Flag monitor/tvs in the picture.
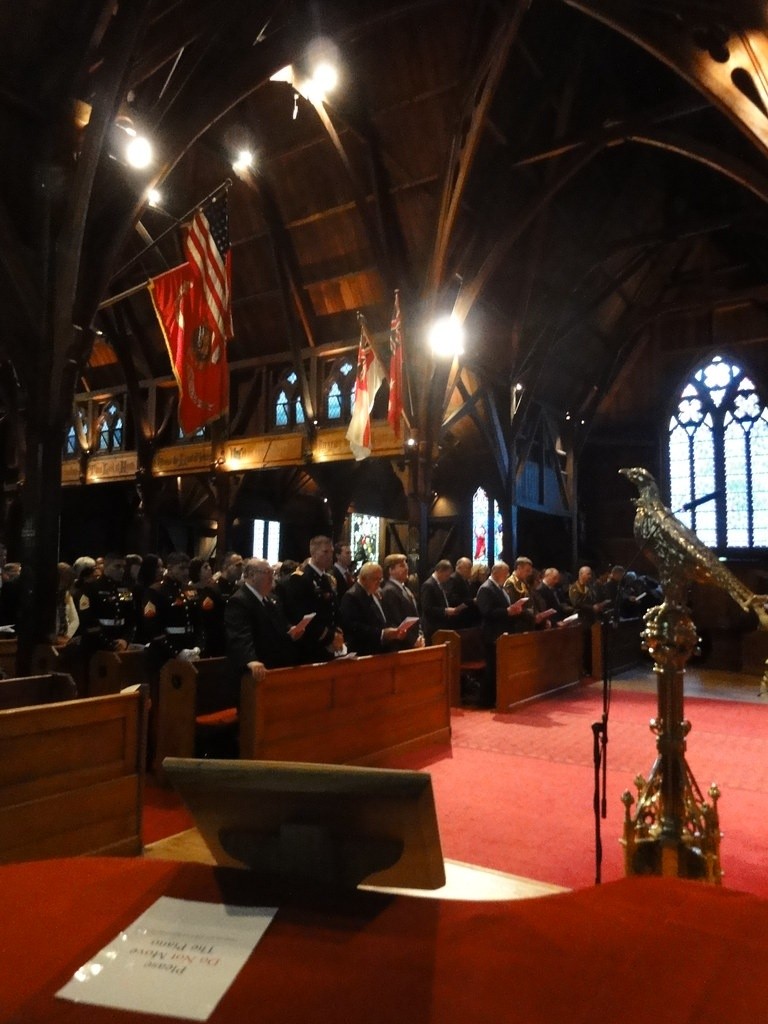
[162,755,447,891]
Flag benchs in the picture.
[0,626,500,786]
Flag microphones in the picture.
[613,489,724,628]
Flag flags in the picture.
[182,185,235,345]
[346,323,387,461]
[386,293,407,439]
[150,257,229,438]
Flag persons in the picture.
[1,534,676,727]
